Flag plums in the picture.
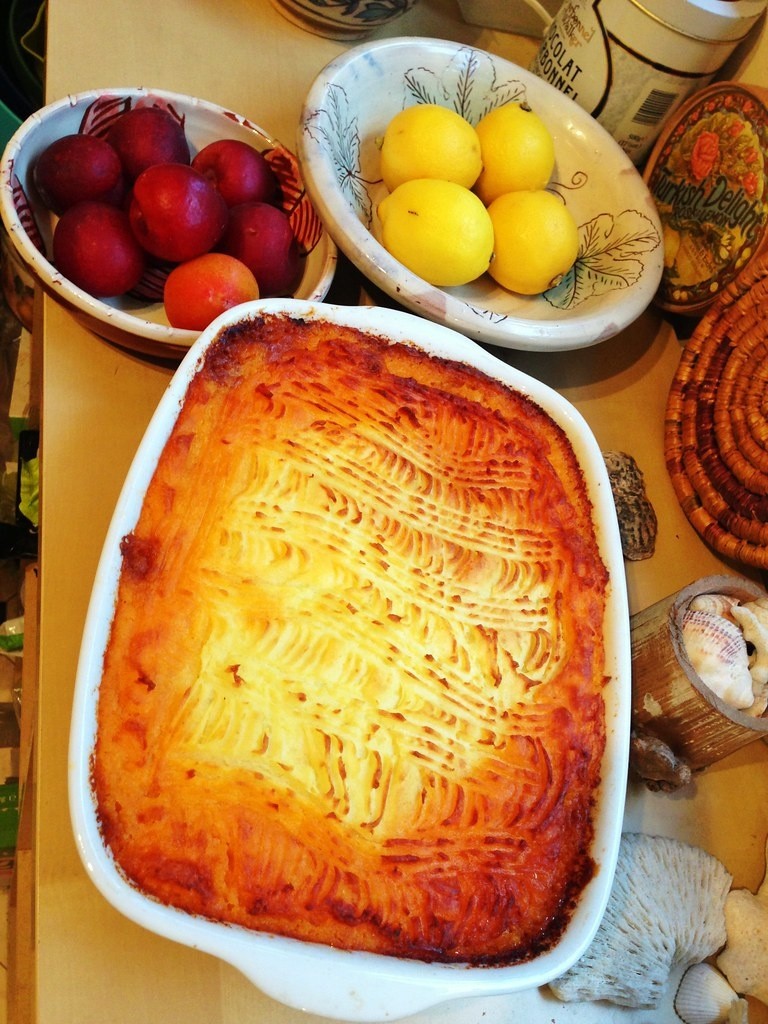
[22,105,296,335]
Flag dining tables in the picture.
[8,0,767,1024]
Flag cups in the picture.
[634,576,768,787]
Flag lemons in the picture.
[371,101,581,292]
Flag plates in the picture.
[63,302,633,1024]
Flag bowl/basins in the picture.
[3,85,336,356]
[305,33,662,350]
